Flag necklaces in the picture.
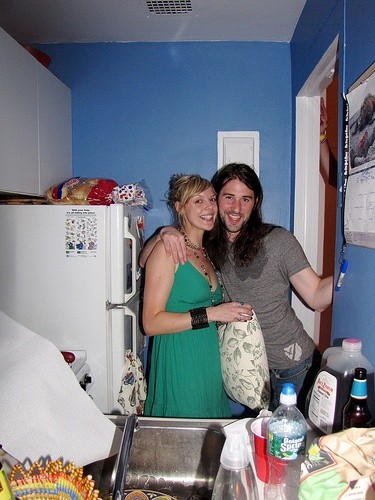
[177,226,225,331]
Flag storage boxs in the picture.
[23,43,53,69]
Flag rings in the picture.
[240,301,244,306]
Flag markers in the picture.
[334,259,349,291]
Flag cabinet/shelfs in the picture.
[0,25,75,200]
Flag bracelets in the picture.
[189,306,210,330]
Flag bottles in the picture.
[265,382,307,500]
[308,339,375,439]
[212,431,260,500]
[342,368,372,432]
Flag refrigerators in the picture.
[0,203,146,417]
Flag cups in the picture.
[250,417,302,485]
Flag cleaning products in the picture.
[212,431,260,500]
[263,382,309,499]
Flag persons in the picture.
[142,173,253,418]
[138,162,335,419]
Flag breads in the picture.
[45,179,98,205]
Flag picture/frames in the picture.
[216,130,259,178]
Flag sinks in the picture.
[83,417,126,500]
[110,416,236,500]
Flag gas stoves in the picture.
[60,350,96,395]
